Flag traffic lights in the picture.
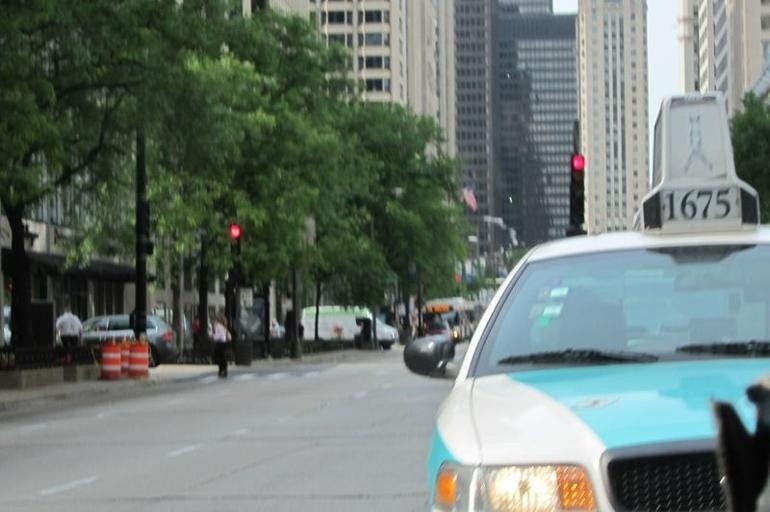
[570,154,584,226]
[227,221,242,266]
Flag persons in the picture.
[443,305,457,332]
[190,314,200,349]
[207,311,234,378]
[56,304,85,362]
[299,323,304,347]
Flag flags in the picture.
[463,184,479,213]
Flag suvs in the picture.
[56,310,180,370]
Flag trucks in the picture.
[298,303,399,351]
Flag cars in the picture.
[403,92,769,512]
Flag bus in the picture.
[419,295,478,344]
